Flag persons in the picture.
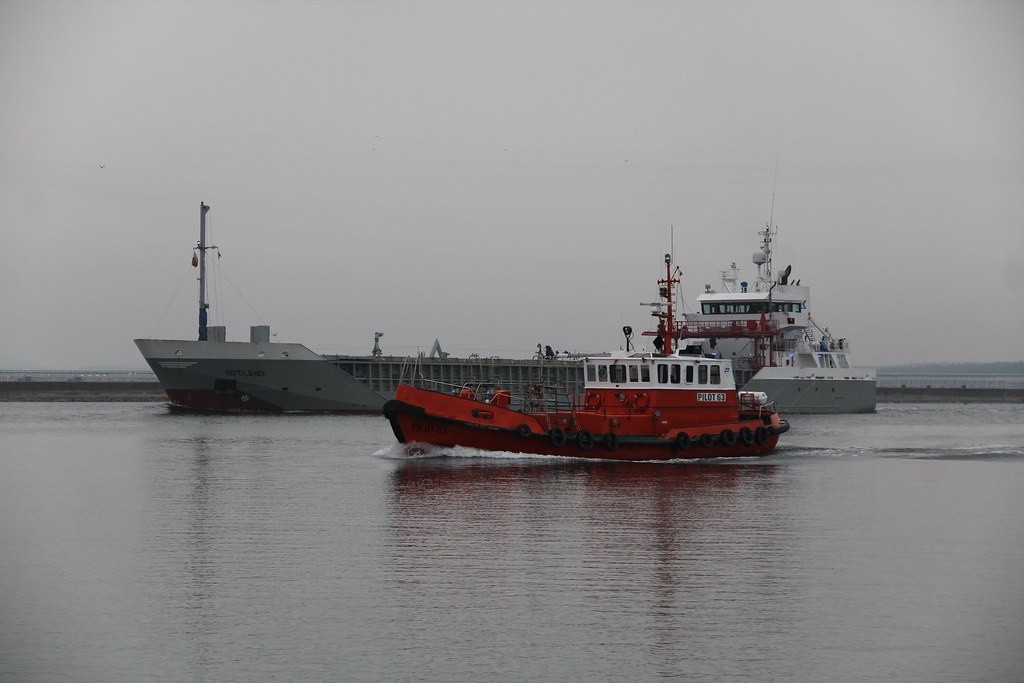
[820,336,846,350]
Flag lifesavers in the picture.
[583,392,602,410]
[632,392,650,412]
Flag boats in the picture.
[379,356,790,459]
[133,224,880,416]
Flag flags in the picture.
[192,252,198,268]
